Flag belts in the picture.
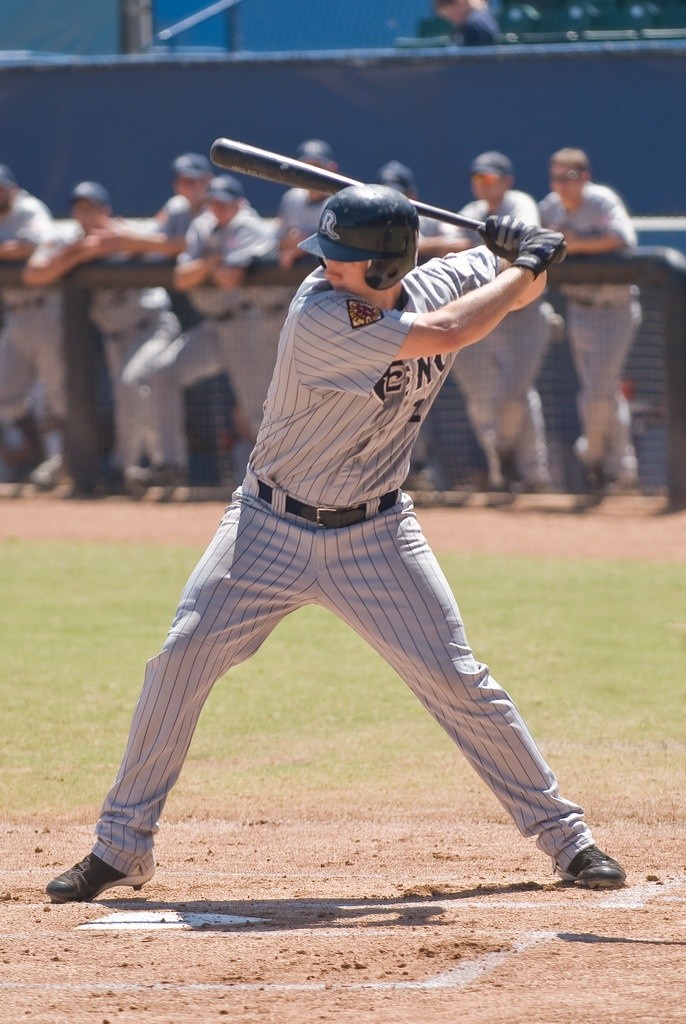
[257,480,399,530]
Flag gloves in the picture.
[477,215,529,263]
[512,224,564,282]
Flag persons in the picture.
[538,149,641,495]
[24,180,189,486]
[0,163,67,487]
[376,159,472,257]
[434,0,501,48]
[146,152,212,262]
[450,150,557,490]
[46,183,626,903]
[278,137,339,268]
[173,174,289,439]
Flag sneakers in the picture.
[45,852,155,903]
[557,846,628,887]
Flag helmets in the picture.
[68,180,110,205]
[297,184,420,290]
[469,151,514,180]
[171,153,211,180]
[377,160,416,194]
[204,173,245,202]
[293,139,336,167]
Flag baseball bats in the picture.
[210,137,568,264]
